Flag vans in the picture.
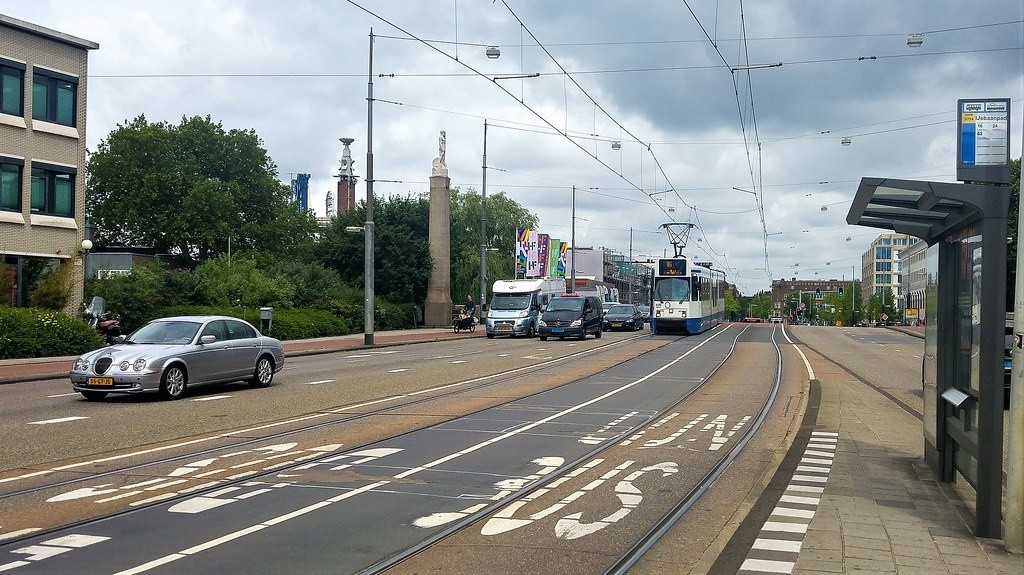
[538,293,604,341]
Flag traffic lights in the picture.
[815,287,821,296]
[796,308,804,317]
[838,287,844,296]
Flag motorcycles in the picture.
[81,296,122,344]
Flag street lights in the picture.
[345,222,374,345]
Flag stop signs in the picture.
[838,306,842,310]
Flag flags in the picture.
[515,227,568,279]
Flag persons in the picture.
[458,294,476,329]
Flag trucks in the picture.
[482,278,567,338]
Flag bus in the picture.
[649,258,726,336]
[743,318,761,323]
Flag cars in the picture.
[638,305,651,323]
[791,320,811,326]
[603,304,644,331]
[68,315,286,401]
[601,302,621,316]
[921,319,1014,411]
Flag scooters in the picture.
[452,308,478,333]
